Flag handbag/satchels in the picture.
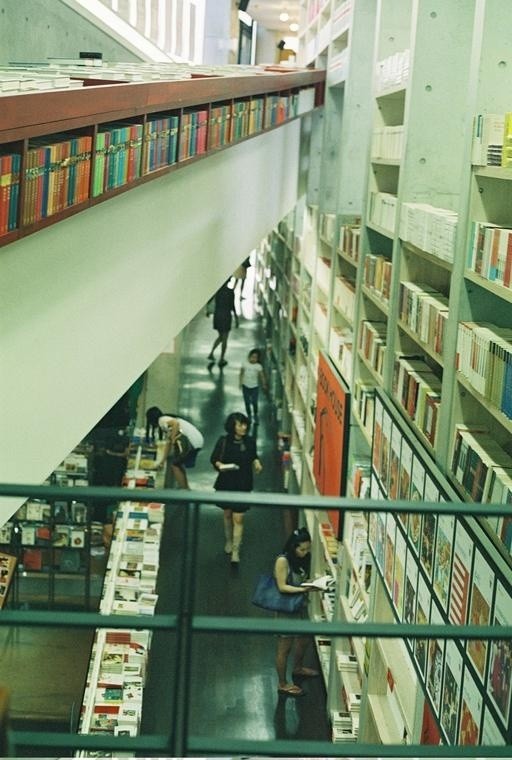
[207,298,218,314]
[251,573,309,614]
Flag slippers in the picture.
[278,682,305,697]
[293,668,321,677]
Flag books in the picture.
[0,428,166,757]
[258,111,511,746]
[297,0,410,90]
[0,59,325,234]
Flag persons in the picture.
[206,278,239,366]
[210,410,321,697]
[146,409,203,490]
[233,256,250,299]
[239,349,266,424]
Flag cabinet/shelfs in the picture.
[0,444,92,612]
[0,65,326,247]
